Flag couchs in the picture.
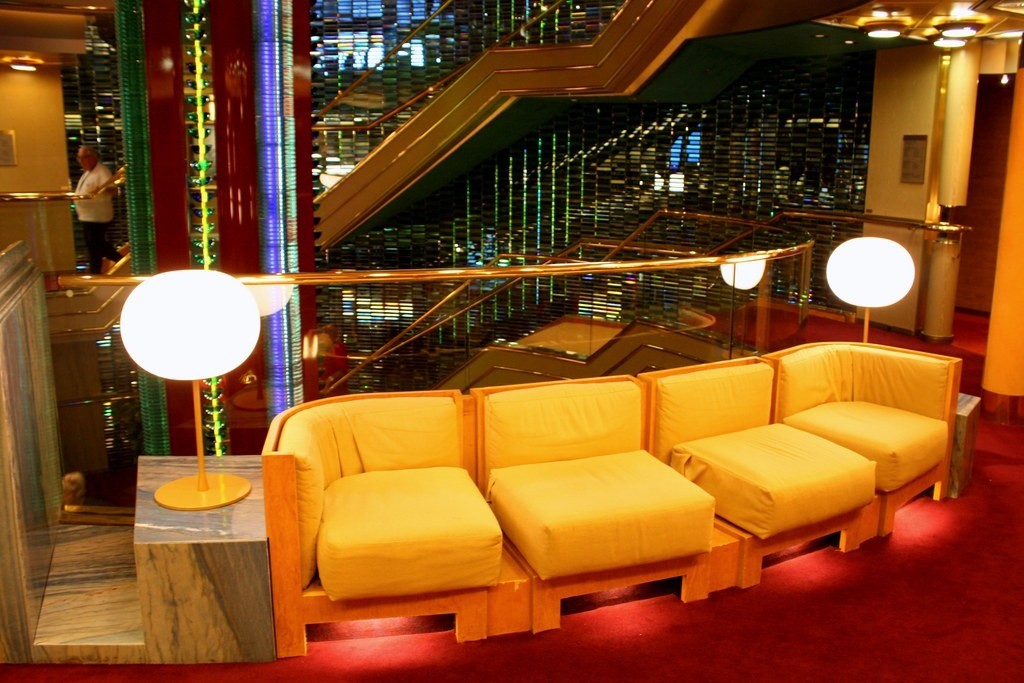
[261,340,963,659]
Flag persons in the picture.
[73,144,123,276]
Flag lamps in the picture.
[826,236,915,344]
[119,269,261,511]
[720,259,768,355]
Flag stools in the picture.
[948,393,982,498]
